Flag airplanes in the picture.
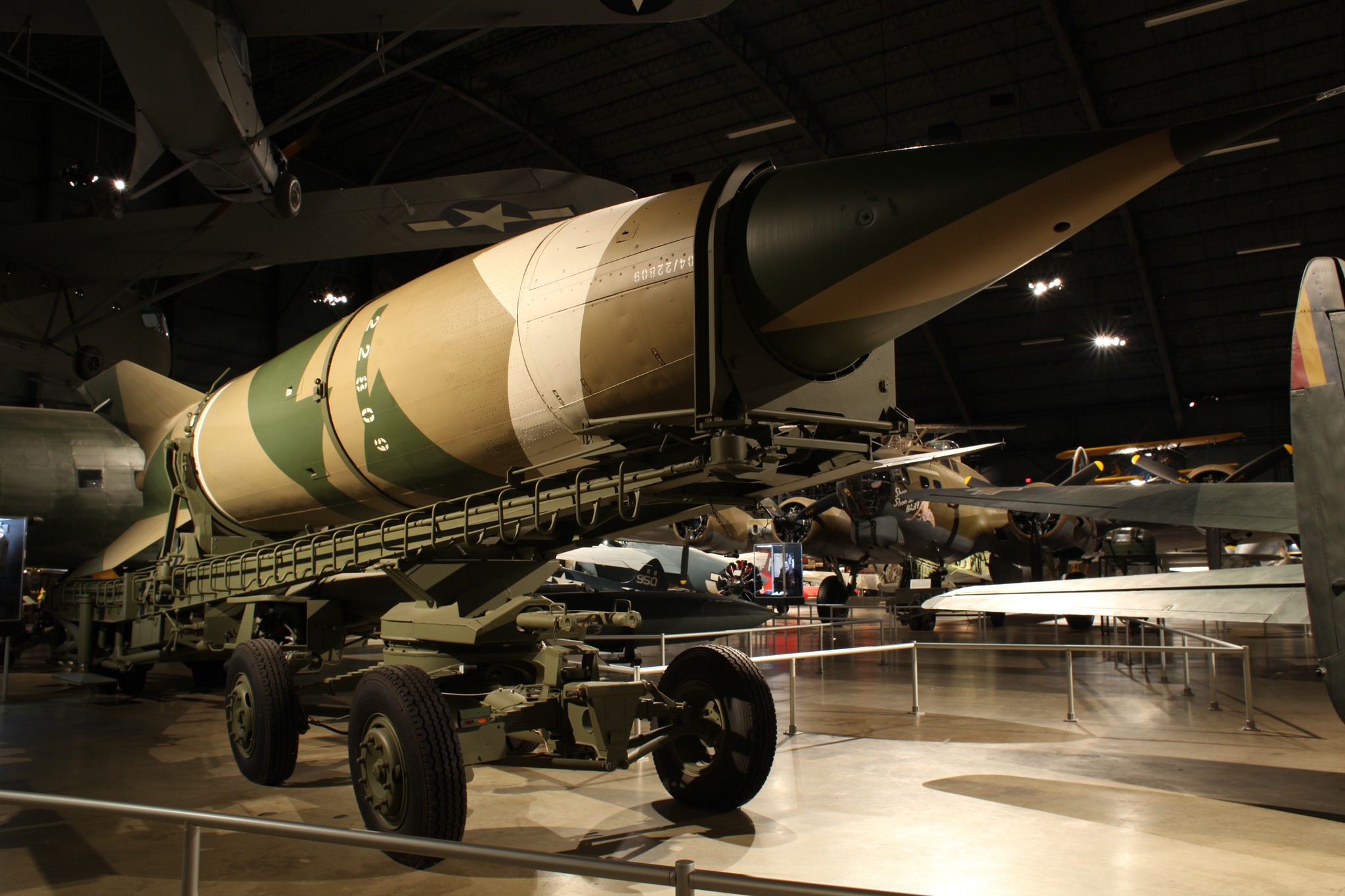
[0,0,1345,873]
